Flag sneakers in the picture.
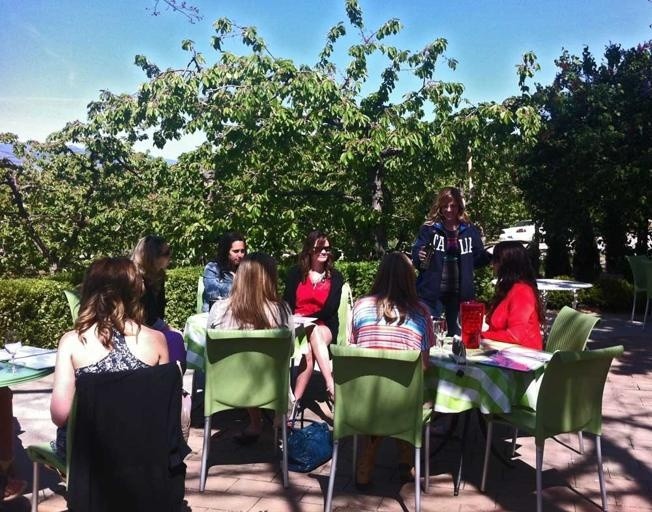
[233,416,283,463]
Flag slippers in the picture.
[0,479,27,502]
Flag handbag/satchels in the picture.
[449,334,466,365]
[287,422,332,473]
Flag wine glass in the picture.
[3,334,22,376]
[432,320,448,353]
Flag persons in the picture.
[451,240,546,438]
[278,230,346,429]
[409,186,493,338]
[129,234,186,374]
[209,253,294,437]
[346,249,438,493]
[2,387,30,505]
[199,231,251,310]
[47,253,171,467]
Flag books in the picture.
[1,343,60,372]
[482,343,552,375]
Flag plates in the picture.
[498,238,531,244]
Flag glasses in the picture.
[313,247,330,251]
[159,247,173,258]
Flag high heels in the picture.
[325,388,335,413]
[288,399,301,429]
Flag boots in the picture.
[356,435,381,484]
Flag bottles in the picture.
[420,231,436,270]
